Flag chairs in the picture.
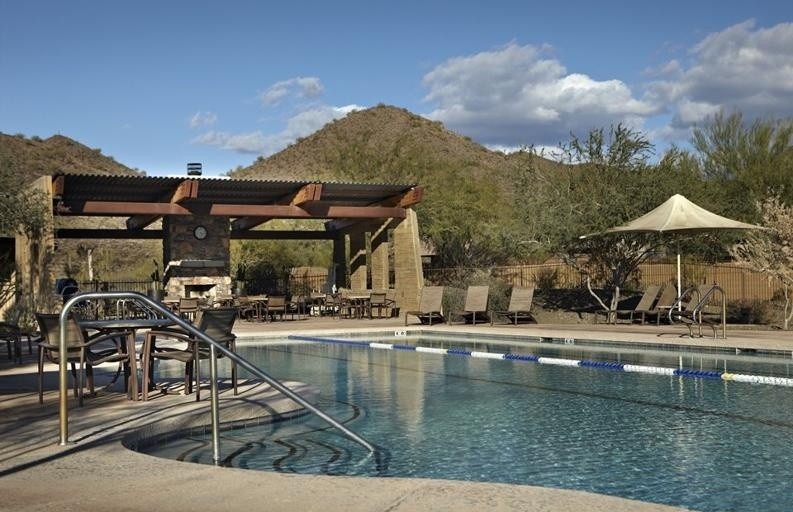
[138,305,242,403]
[447,285,492,329]
[33,310,134,406]
[56,278,87,307]
[2,287,45,365]
[594,286,713,341]
[403,285,448,327]
[494,284,539,328]
[114,290,399,324]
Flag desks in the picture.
[81,316,179,400]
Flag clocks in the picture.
[193,226,208,240]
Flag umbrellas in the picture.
[578,193,771,322]
